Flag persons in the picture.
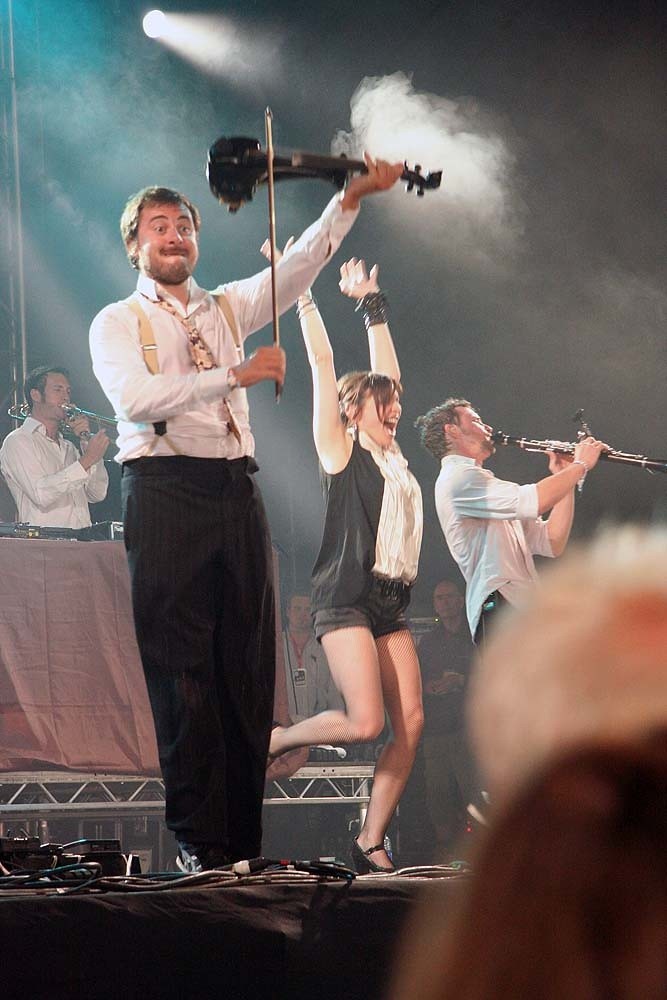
[88,152,405,878]
[243,235,428,874]
[0,367,111,529]
[284,396,667,1000]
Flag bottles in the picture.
[384,835,393,862]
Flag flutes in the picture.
[492,427,667,471]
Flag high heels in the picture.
[351,836,395,874]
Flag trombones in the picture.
[5,399,118,432]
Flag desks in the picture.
[0,540,308,783]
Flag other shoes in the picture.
[175,842,233,876]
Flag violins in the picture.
[207,134,446,215]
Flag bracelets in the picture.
[352,293,388,330]
[572,461,587,492]
[295,295,318,318]
